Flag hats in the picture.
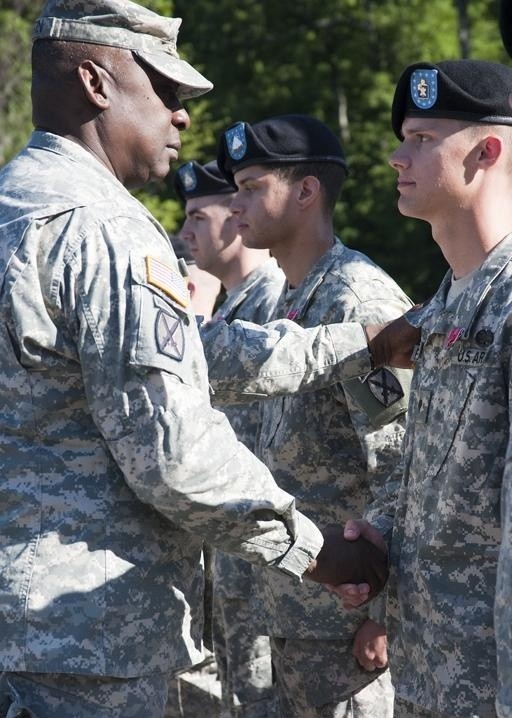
[392,60,512,142]
[33,0,214,100]
[174,114,345,202]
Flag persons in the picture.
[0,0,423,718]
[167,115,416,718]
[322,59,512,718]
